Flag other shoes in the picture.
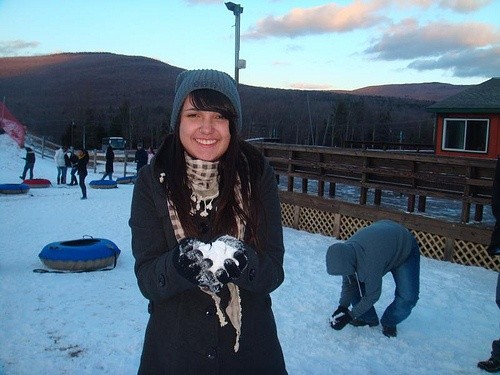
[383,324,398,339]
[81,196,86,200]
[73,183,78,186]
[20,176,24,180]
[67,183,74,186]
[351,316,377,327]
[479,357,500,372]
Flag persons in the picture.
[134,143,148,176]
[148,146,155,165]
[20,147,35,180]
[101,147,114,180]
[326,219,420,338]
[77,148,89,199]
[477,159,500,373]
[54,145,70,184]
[70,153,79,184]
[129,70,289,375]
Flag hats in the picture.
[170,68,241,136]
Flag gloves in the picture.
[330,306,350,329]
[174,239,213,285]
[216,253,246,284]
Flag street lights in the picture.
[224,1,246,91]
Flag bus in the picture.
[102,136,127,153]
